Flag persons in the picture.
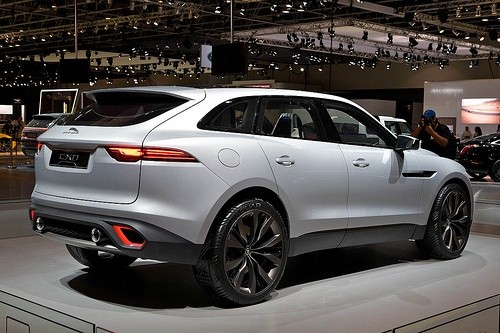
[413,110,456,161]
[461,127,473,140]
[2,116,24,138]
[473,127,482,138]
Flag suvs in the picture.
[28,86,474,306]
[21,114,61,158]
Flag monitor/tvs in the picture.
[201,45,212,68]
[59,58,89,83]
[212,42,248,76]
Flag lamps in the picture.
[0,0,500,92]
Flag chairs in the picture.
[237,112,305,140]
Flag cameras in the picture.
[422,118,432,126]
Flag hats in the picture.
[421,110,436,118]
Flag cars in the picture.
[457,132,500,183]
